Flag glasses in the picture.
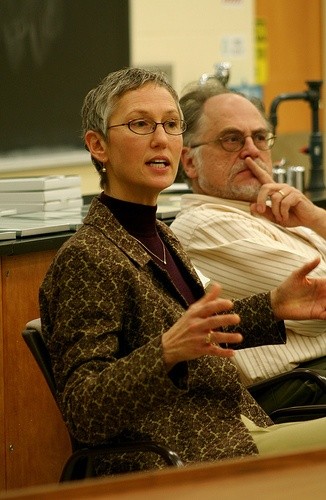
[191,131,277,153]
[107,117,190,136]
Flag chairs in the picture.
[21,315,185,479]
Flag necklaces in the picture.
[125,224,167,265]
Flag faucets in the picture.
[267,79,325,191]
[198,63,231,89]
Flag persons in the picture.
[38,67,326,478]
[168,81,326,423]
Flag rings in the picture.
[278,190,285,198]
[205,330,215,345]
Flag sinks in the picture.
[81,193,182,214]
